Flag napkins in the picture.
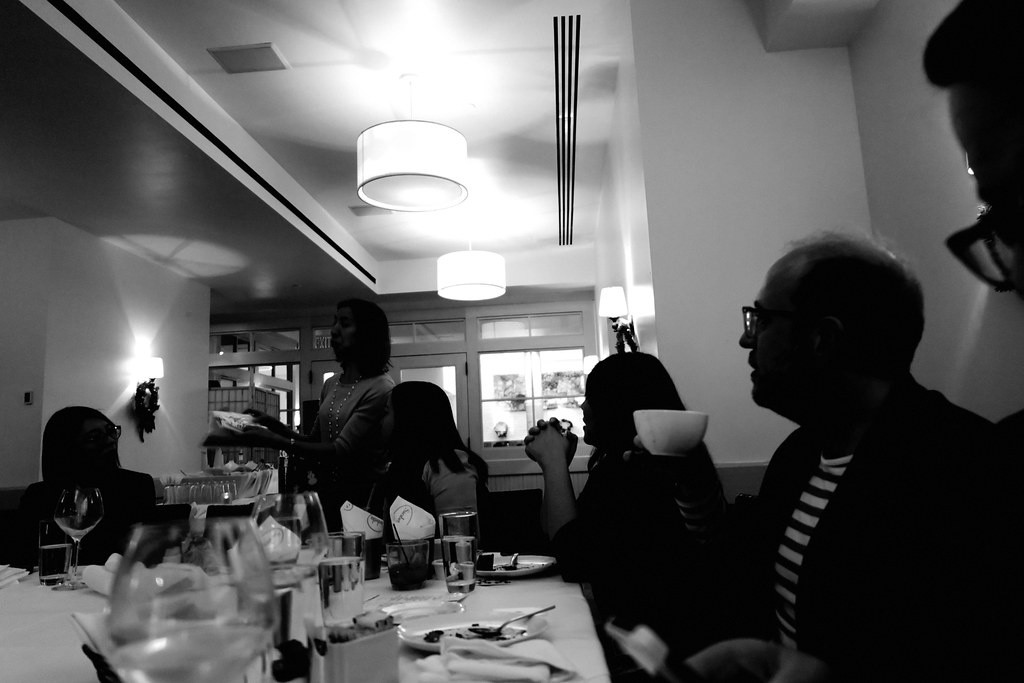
[0,563,30,590]
[413,636,581,682]
[71,583,238,659]
[258,515,301,563]
[84,553,124,597]
[389,495,436,540]
[340,500,384,540]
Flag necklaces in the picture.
[328,375,362,438]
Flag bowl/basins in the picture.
[633,409,709,457]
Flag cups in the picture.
[39,516,73,587]
[439,511,479,594]
[310,531,366,635]
[385,540,430,591]
[164,479,240,504]
[251,491,332,590]
[321,557,366,633]
[101,516,280,683]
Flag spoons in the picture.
[502,553,519,571]
[467,604,556,636]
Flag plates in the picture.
[476,556,555,579]
[382,601,463,625]
[398,610,547,652]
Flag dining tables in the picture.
[0,567,610,683]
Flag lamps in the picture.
[356,73,470,212]
[437,239,506,301]
[598,285,634,337]
[133,357,163,415]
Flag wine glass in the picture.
[52,485,104,591]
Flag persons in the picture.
[525,352,731,670]
[0,406,156,572]
[623,233,995,667]
[381,382,489,543]
[686,0,1024,683]
[236,299,396,532]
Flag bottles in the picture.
[237,453,245,466]
[252,459,264,472]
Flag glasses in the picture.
[742,306,823,338]
[74,425,122,446]
[946,202,1023,290]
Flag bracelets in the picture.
[285,438,296,456]
